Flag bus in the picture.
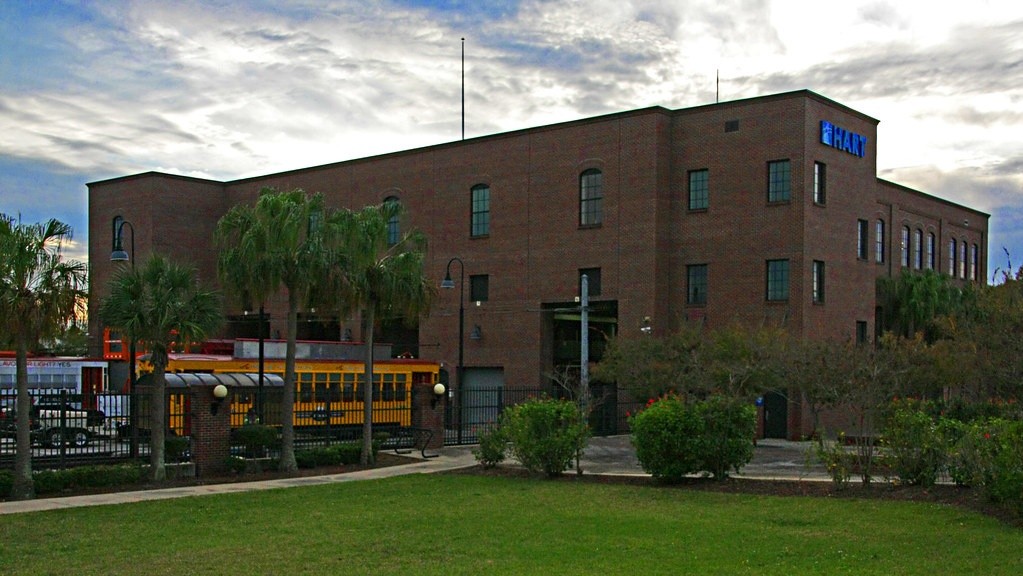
[129,337,442,443]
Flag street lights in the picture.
[440,257,473,445]
[109,221,139,461]
[575,274,588,424]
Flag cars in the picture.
[0,394,119,448]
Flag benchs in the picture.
[838,435,900,476]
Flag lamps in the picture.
[470,324,482,340]
[431,383,445,410]
[210,385,227,416]
